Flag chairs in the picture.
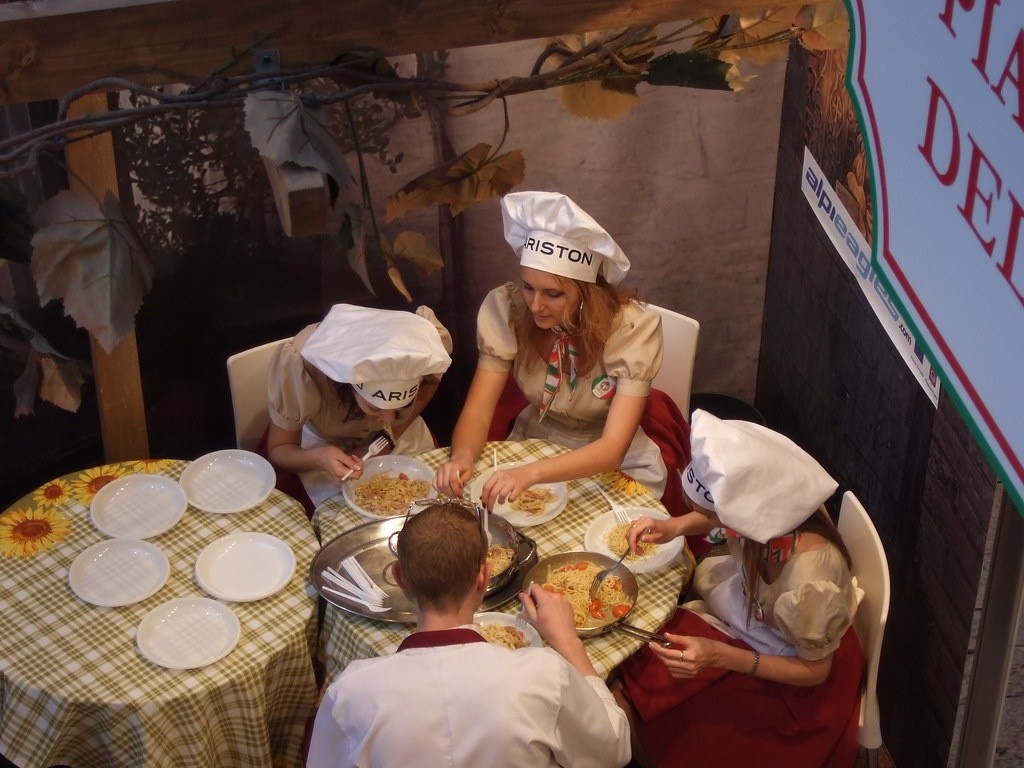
[836,490,891,768]
[225,336,295,452]
[625,296,699,424]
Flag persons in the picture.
[308,503,633,767]
[433,191,691,515]
[254,303,452,518]
[609,409,868,768]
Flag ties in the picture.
[703,527,797,574]
[538,324,579,424]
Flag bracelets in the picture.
[375,433,395,449]
[752,652,759,673]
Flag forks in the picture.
[482,505,492,548]
[342,436,388,481]
[596,484,629,523]
[590,533,644,600]
[322,556,392,612]
[517,579,534,629]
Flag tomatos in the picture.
[513,631,524,640]
[398,473,408,480]
[542,562,632,620]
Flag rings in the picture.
[633,524,637,528]
[681,650,682,660]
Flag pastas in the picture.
[541,560,622,626]
[511,488,553,516]
[478,622,532,650]
[354,473,429,516]
[485,543,513,577]
[607,520,658,561]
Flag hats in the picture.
[500,191,630,284]
[302,305,452,409]
[680,407,841,542]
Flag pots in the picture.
[522,552,672,650]
[388,515,536,595]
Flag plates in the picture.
[471,613,543,649]
[585,507,684,574]
[471,460,568,526]
[180,450,276,514]
[91,475,187,541]
[137,598,240,669]
[342,456,438,519]
[68,540,170,606]
[195,532,296,602]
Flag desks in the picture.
[0,458,322,768]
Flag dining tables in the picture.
[310,439,698,715]
